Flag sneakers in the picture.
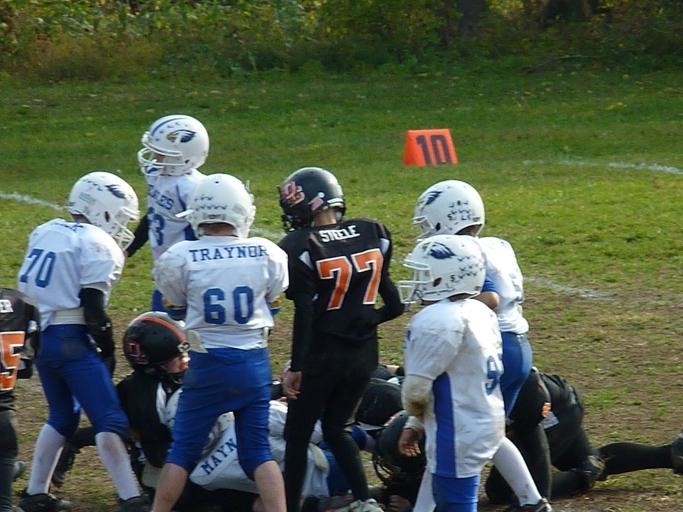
[111,492,151,512]
[332,497,384,511]
[669,433,683,474]
[575,455,607,490]
[496,496,554,512]
[15,481,74,510]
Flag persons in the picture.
[1,114,682,511]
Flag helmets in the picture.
[136,114,209,178]
[395,233,487,312]
[275,167,345,235]
[122,311,191,388]
[410,179,484,242]
[349,382,425,490]
[62,171,141,252]
[185,173,256,239]
